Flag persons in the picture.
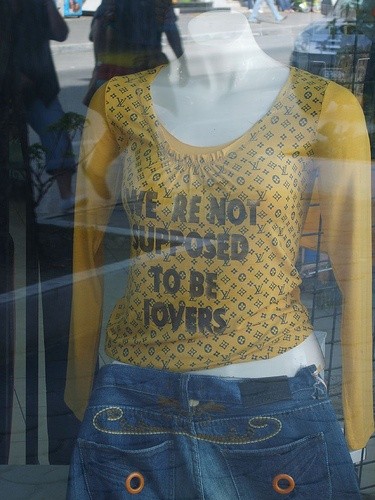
[84,0,190,123]
[0,0,80,216]
[248,0,296,23]
[65,11,373,500]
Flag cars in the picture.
[287,0,375,85]
